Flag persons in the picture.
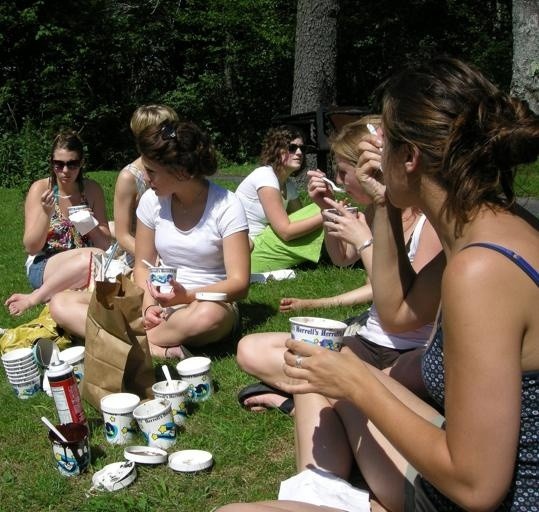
[234,124,352,274]
[4,133,112,316]
[101,103,255,272]
[48,120,249,361]
[212,57,539,511]
[234,113,444,419]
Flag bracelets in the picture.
[356,238,372,256]
[144,303,157,317]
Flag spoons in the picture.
[319,176,347,193]
[53,193,73,200]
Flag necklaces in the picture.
[173,184,208,215]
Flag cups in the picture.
[49,423,89,478]
[324,205,362,233]
[59,346,85,389]
[148,267,178,287]
[2,348,40,400]
[65,205,87,217]
[100,356,214,450]
[288,316,348,356]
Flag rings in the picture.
[295,356,302,369]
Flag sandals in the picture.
[237,381,294,419]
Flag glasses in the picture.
[160,118,185,153]
[286,144,308,156]
[51,159,81,171]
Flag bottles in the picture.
[46,346,92,443]
